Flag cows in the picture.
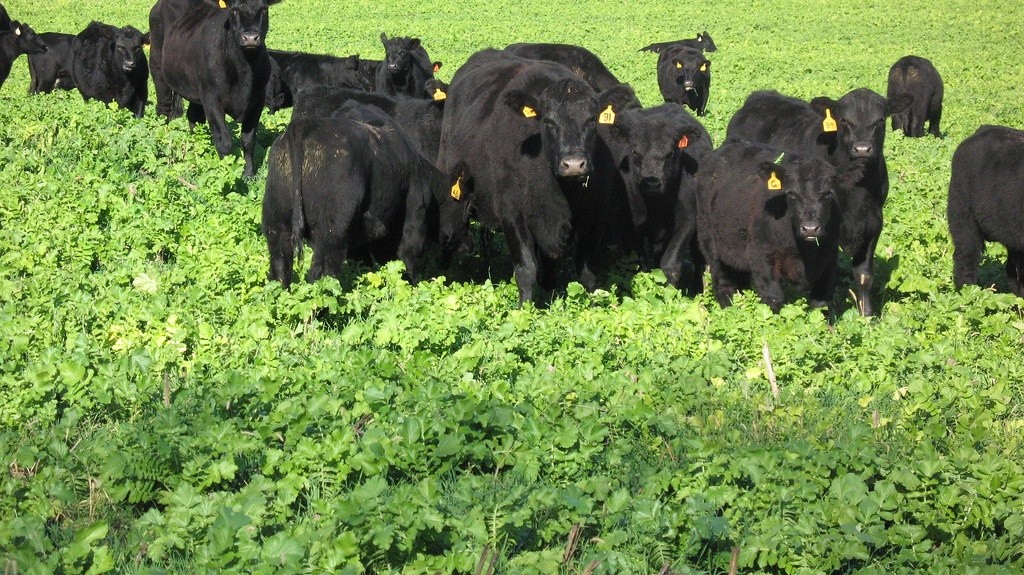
[945,123,1024,297]
[1,0,916,322]
[885,54,945,139]
